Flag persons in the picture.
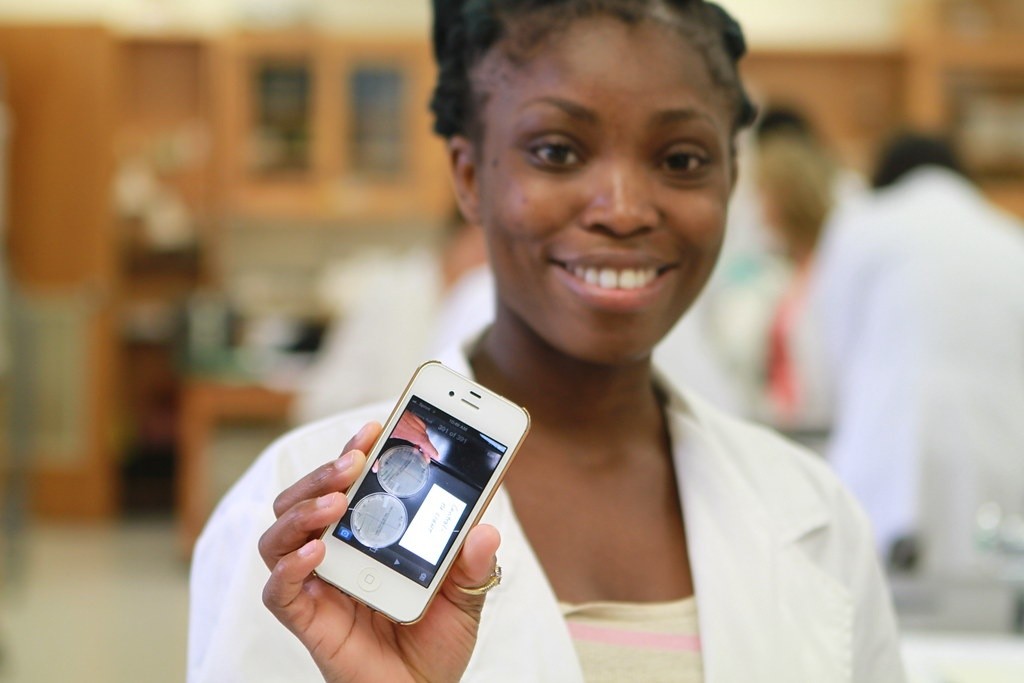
[188,1,908,683]
[287,84,1024,633]
[372,409,439,473]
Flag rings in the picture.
[447,566,503,596]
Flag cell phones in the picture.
[312,360,532,626]
